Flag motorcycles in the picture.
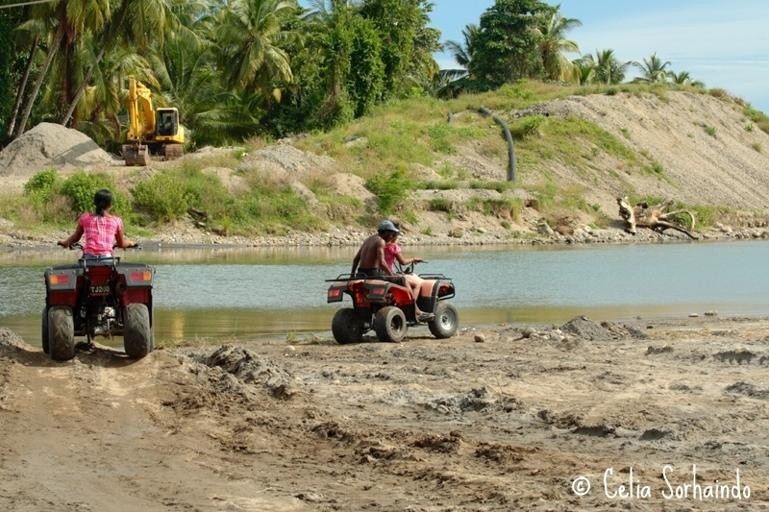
[324,259,458,342]
[41,241,153,359]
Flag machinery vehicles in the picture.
[121,76,184,165]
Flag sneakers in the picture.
[417,312,433,320]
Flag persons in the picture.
[159,116,172,135]
[57,189,137,330]
[381,228,425,303]
[352,221,433,321]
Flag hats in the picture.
[377,220,399,233]
